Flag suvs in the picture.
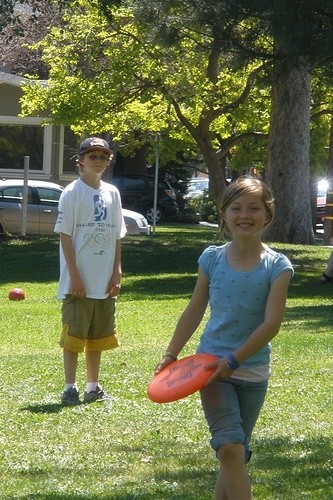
[110,174,178,224]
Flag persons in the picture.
[153,174,295,496]
[53,135,130,408]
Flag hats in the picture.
[80,137,113,154]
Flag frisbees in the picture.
[148,354,220,402]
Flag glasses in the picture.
[89,155,108,161]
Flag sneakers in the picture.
[84,385,113,403]
[62,386,81,405]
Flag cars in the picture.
[183,179,233,201]
[0,179,150,237]
[315,180,332,223]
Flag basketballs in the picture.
[8,289,25,301]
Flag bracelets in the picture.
[223,351,243,375]
[162,350,180,362]
